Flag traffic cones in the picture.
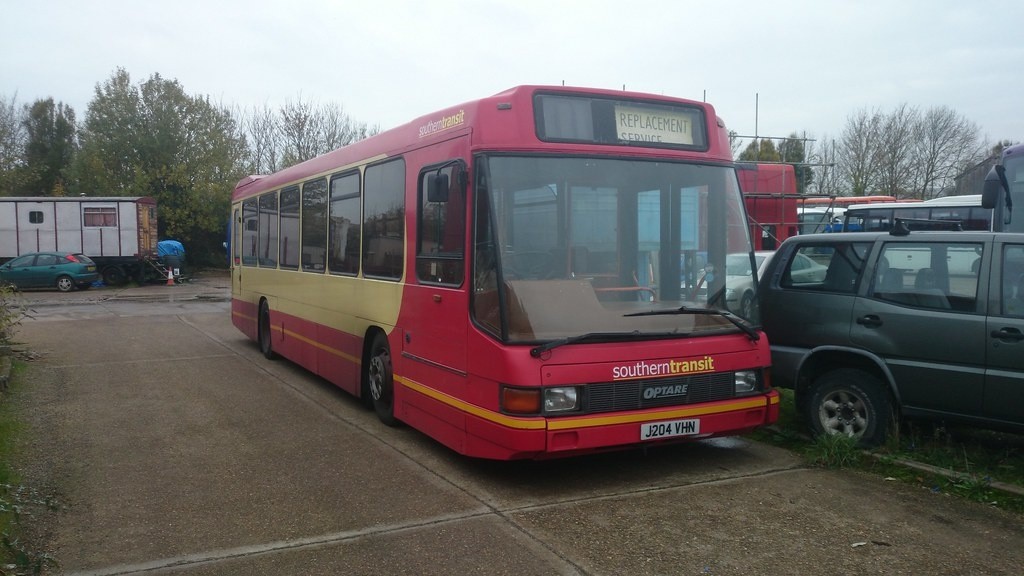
[165,266,176,285]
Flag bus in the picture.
[226,84,784,464]
[754,218,1024,450]
[798,196,925,208]
[849,195,994,232]
[797,207,847,234]
[981,143,1024,232]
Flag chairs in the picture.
[879,268,918,306]
[583,247,624,303]
[911,267,951,310]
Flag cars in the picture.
[679,251,830,322]
[0,251,97,291]
